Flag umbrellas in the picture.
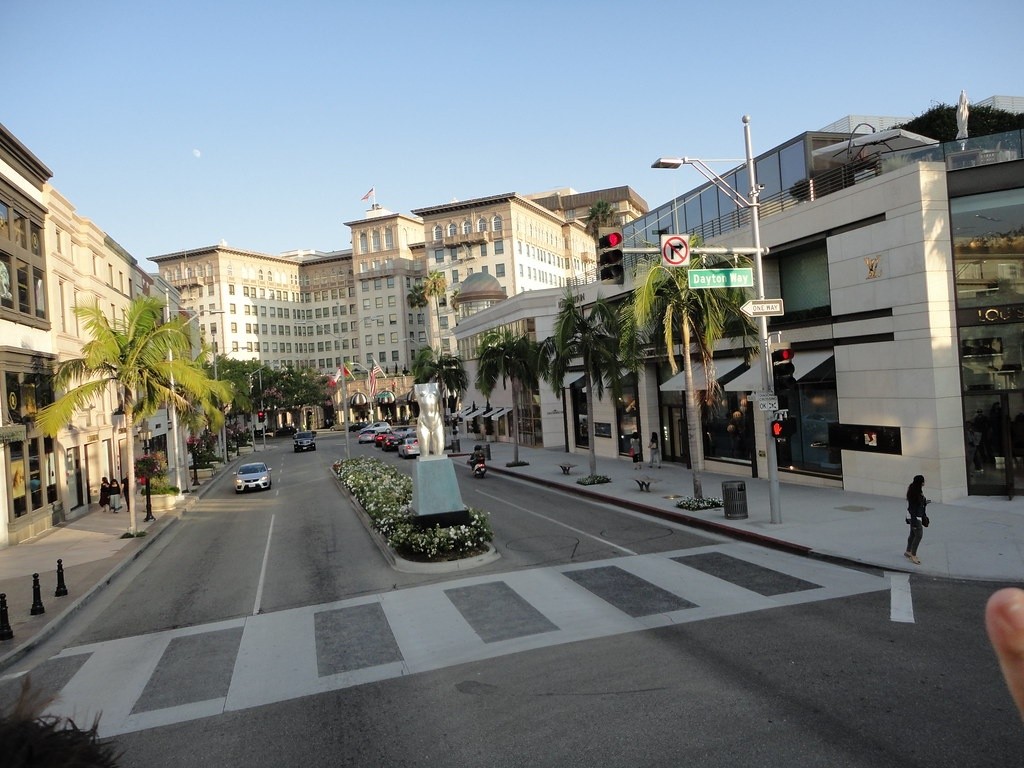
[811,128,939,178]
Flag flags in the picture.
[361,189,374,202]
[369,360,382,396]
[329,364,349,387]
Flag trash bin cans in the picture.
[452,439,460,453]
[482,444,491,460]
[722,481,748,520]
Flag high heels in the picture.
[904,551,920,564]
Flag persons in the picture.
[98,471,129,513]
[581,418,588,445]
[325,415,411,428]
[905,475,932,564]
[469,445,485,471]
[630,432,642,470]
[965,402,1024,474]
[984,588,1024,720]
[648,432,661,468]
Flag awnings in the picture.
[563,368,634,392]
[350,389,419,405]
[452,407,512,421]
[660,349,834,391]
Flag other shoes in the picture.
[633,468,636,470]
[648,466,652,468]
[657,466,661,469]
[974,469,983,472]
[639,464,641,470]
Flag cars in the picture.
[293,431,317,452]
[330,421,420,459]
[233,462,273,494]
[250,426,297,436]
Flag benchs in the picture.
[627,474,661,492]
[555,461,577,475]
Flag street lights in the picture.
[248,354,299,452]
[294,315,383,458]
[350,362,373,424]
[651,156,782,523]
[165,308,225,501]
[401,337,431,349]
[138,418,156,522]
[211,346,249,464]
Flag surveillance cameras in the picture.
[755,184,765,190]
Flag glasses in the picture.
[977,411,982,413]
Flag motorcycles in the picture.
[468,452,487,479]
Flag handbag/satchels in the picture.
[630,448,634,457]
[649,442,656,449]
[906,519,910,524]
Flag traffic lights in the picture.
[774,348,797,390]
[258,410,265,422]
[602,232,623,279]
[771,420,786,438]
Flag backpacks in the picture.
[970,431,982,445]
